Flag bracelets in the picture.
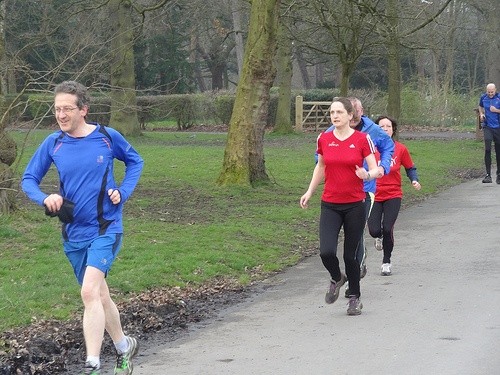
[367,171,371,180]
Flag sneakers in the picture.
[482,173,492,183]
[380,262,392,277]
[82,359,101,375]
[346,297,364,316]
[113,334,140,375]
[325,274,348,304]
[495,172,500,185]
[374,237,384,251]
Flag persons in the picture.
[478,82,500,185]
[20,81,143,375]
[300,98,376,316]
[367,114,421,276]
[315,97,395,279]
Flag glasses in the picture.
[55,106,80,112]
